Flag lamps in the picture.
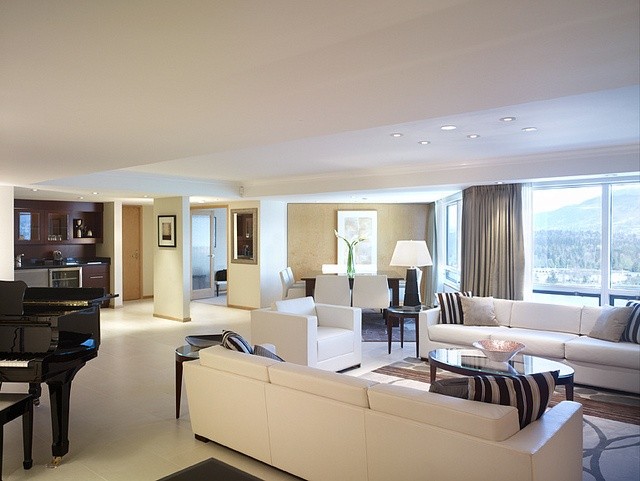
[388,240,434,307]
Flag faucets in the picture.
[16,254,24,264]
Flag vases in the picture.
[346,245,356,275]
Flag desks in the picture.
[385,305,433,358]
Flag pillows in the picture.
[427,377,468,400]
[459,296,501,326]
[467,369,561,431]
[253,344,286,364]
[434,291,475,325]
[619,301,640,345]
[587,303,635,343]
[221,330,254,355]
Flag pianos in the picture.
[0,280,119,468]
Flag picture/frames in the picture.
[157,215,177,247]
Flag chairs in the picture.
[214,270,227,298]
[351,274,391,309]
[279,266,306,298]
[313,274,352,307]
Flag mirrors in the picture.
[230,208,258,265]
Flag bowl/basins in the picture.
[472,339,525,362]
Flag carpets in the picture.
[356,355,640,481]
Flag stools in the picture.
[1,392,35,481]
[175,343,202,419]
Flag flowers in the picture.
[333,229,371,273]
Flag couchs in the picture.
[181,343,640,481]
[418,295,640,394]
[250,295,363,372]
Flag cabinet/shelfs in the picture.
[14,198,104,246]
[82,262,110,308]
